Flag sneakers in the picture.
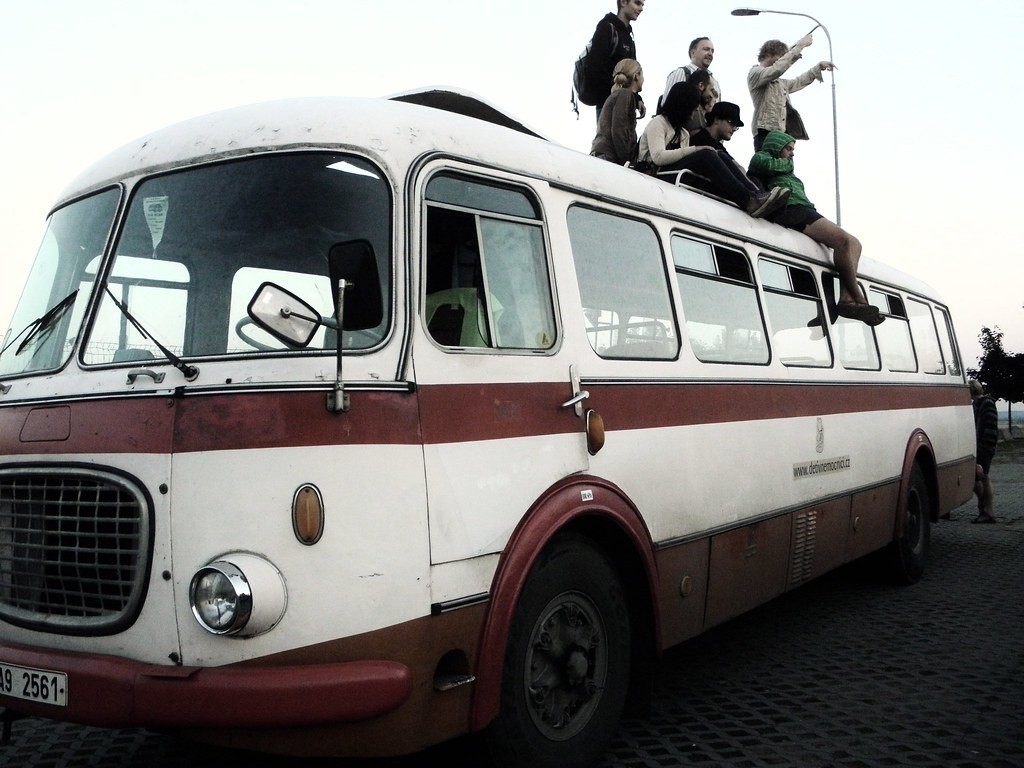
[759,187,791,218]
[746,186,781,218]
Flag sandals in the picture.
[864,314,885,326]
[971,512,996,523]
[836,301,879,322]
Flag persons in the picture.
[590,0,886,327]
[969,380,998,525]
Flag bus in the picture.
[0,89,976,768]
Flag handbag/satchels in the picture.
[633,162,657,177]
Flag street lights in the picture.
[731,8,841,229]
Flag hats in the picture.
[705,102,744,127]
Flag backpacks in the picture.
[573,22,619,106]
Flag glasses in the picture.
[725,118,740,128]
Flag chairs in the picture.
[424,285,488,347]
